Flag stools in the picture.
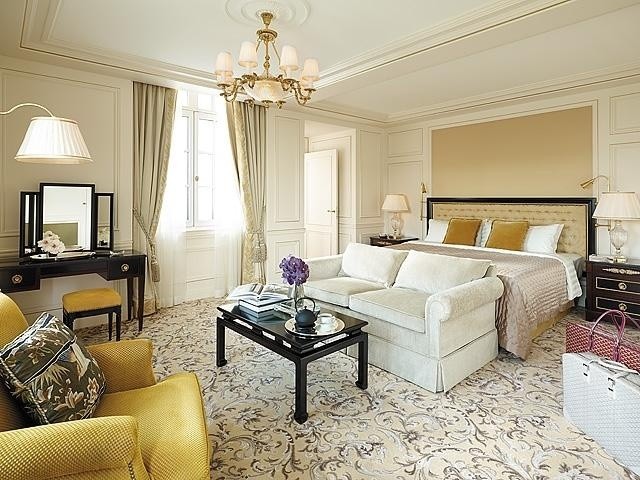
[61,288,123,341]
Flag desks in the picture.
[0,249,148,332]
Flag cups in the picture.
[389,235,393,239]
[396,235,400,239]
[320,313,335,324]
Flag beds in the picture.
[378,197,595,361]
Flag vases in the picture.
[287,284,304,307]
[49,252,58,257]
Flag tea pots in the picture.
[295,297,320,327]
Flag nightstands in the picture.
[583,257,640,329]
[370,236,419,247]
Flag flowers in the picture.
[279,254,309,286]
[43,230,61,240]
[38,238,65,254]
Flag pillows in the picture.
[424,218,565,254]
[0,310,107,425]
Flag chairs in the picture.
[0,293,213,480]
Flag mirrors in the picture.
[19,182,113,264]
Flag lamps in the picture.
[381,194,409,239]
[0,102,94,167]
[420,183,427,220]
[214,10,319,109]
[591,190,640,262]
[580,175,611,231]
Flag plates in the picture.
[379,235,387,239]
[285,316,345,336]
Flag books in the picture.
[225,280,293,323]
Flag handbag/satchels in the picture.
[565,309,639,373]
[562,351,640,475]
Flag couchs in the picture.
[300,240,505,395]
